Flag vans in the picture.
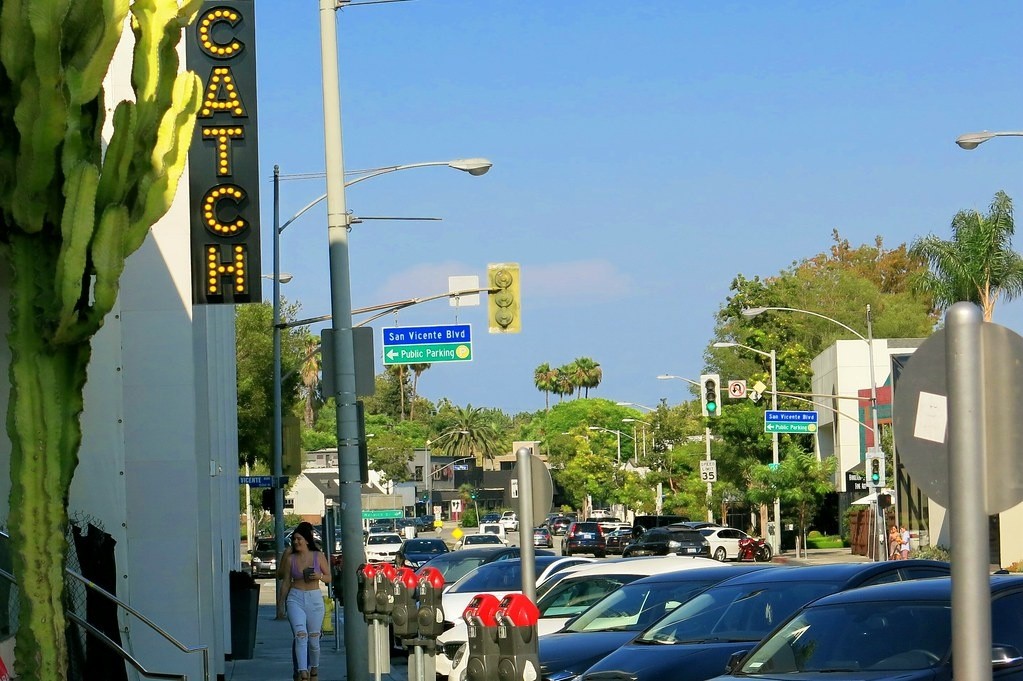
[475,524,507,541]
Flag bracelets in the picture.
[321,576,322,579]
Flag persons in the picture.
[276,522,332,681]
[889,525,910,560]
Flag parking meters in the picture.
[356,562,445,681]
[461,593,503,681]
[496,592,542,681]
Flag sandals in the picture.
[301,672,309,681]
[309,669,317,681]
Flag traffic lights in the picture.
[878,494,891,508]
[866,452,886,487]
[764,421,818,434]
[700,375,720,417]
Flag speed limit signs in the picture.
[699,460,717,482]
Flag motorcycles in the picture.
[736,533,772,561]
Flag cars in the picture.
[450,533,510,550]
[536,564,802,681]
[622,526,713,559]
[695,526,758,562]
[363,531,405,566]
[389,539,451,572]
[571,559,953,681]
[247,513,437,579]
[533,528,554,549]
[537,511,720,558]
[411,546,558,609]
[434,555,731,681]
[703,570,1023,681]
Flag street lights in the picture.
[714,341,782,556]
[589,426,620,465]
[621,418,655,464]
[615,402,663,515]
[274,159,495,616]
[656,373,714,523]
[739,301,890,561]
